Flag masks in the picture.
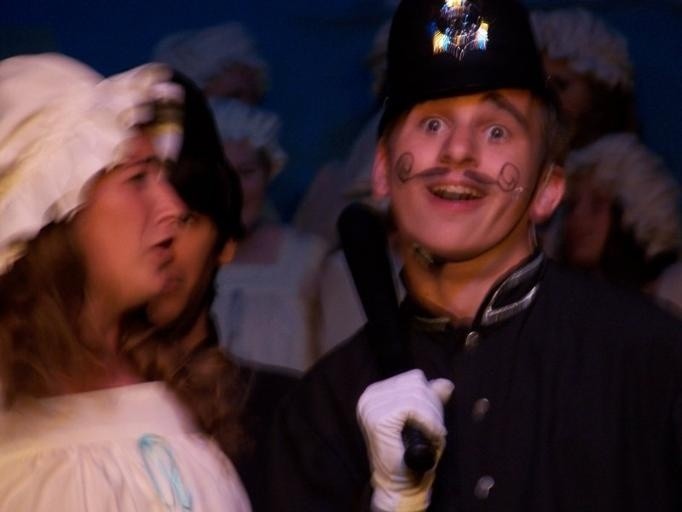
[166,24,267,88]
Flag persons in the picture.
[0,2,682,510]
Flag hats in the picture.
[1,50,175,269]
[210,98,292,175]
[528,7,639,85]
[379,1,549,138]
[161,66,250,240]
[565,134,680,259]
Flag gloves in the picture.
[355,368,455,512]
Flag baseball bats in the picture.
[336,202,436,471]
